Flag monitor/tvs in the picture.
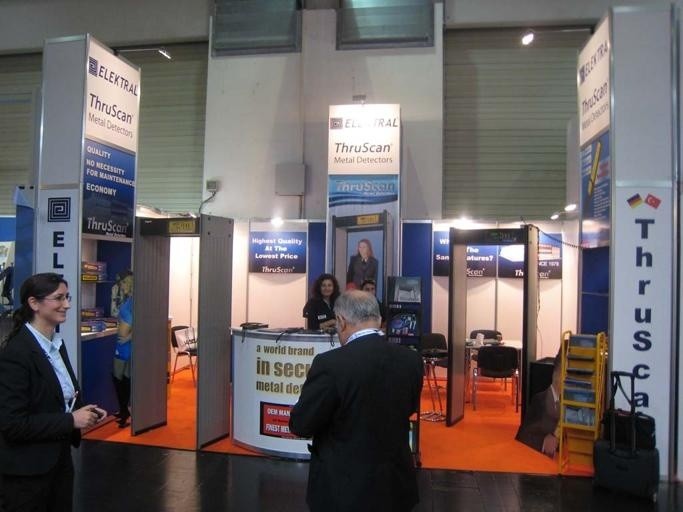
[334,0,435,50]
[210,1,302,54]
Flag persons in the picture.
[0,270,108,511]
[300,274,341,331]
[109,270,135,431]
[285,288,424,511]
[344,238,380,293]
[360,279,387,330]
[513,338,571,460]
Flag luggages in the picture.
[593,372,659,504]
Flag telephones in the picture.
[239,322,268,330]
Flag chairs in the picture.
[171,325,198,388]
[424,330,521,413]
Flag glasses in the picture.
[41,295,72,302]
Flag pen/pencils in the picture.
[66,391,79,413]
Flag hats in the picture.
[115,271,132,284]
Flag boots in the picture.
[110,373,131,425]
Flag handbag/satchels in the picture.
[604,408,656,450]
